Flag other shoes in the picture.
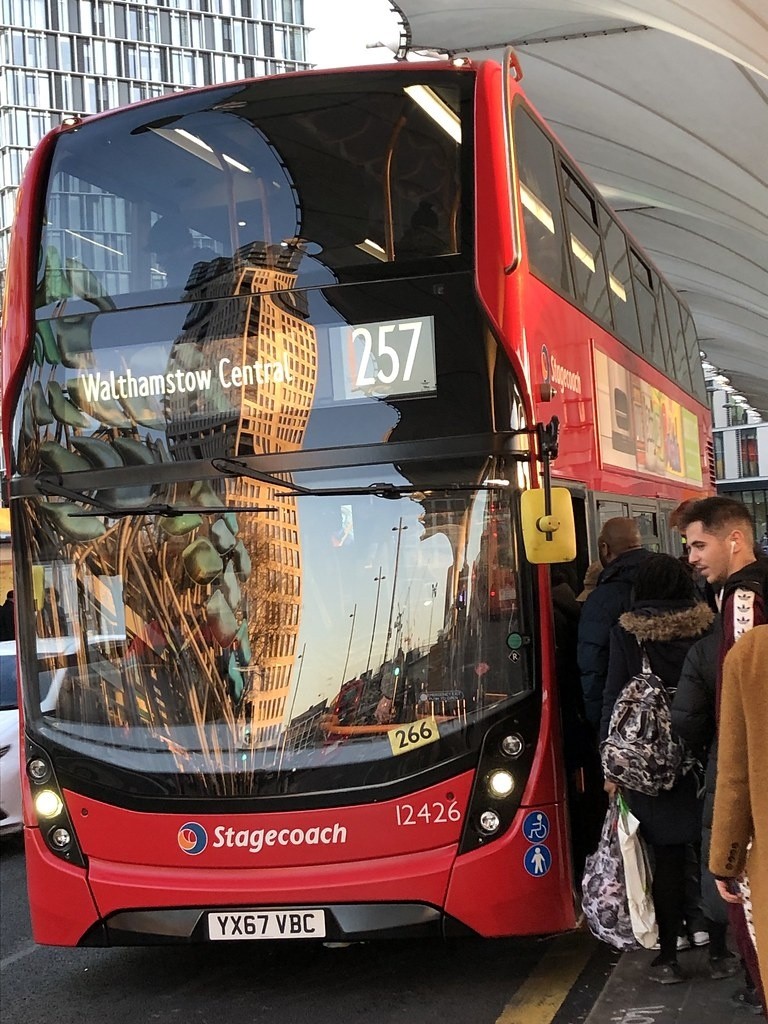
[706,954,739,980]
[731,993,763,1014]
[644,959,690,984]
[674,929,710,950]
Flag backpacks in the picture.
[597,666,682,799]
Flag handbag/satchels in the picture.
[581,797,659,951]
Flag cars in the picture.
[0,635,134,839]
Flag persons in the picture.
[41,587,69,638]
[320,658,411,742]
[116,578,240,661]
[574,496,768,1024]
[0,590,16,642]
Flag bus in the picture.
[0,39,723,950]
[310,676,365,766]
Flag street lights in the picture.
[335,604,355,719]
[358,566,385,712]
[380,518,409,691]
[273,639,306,795]
